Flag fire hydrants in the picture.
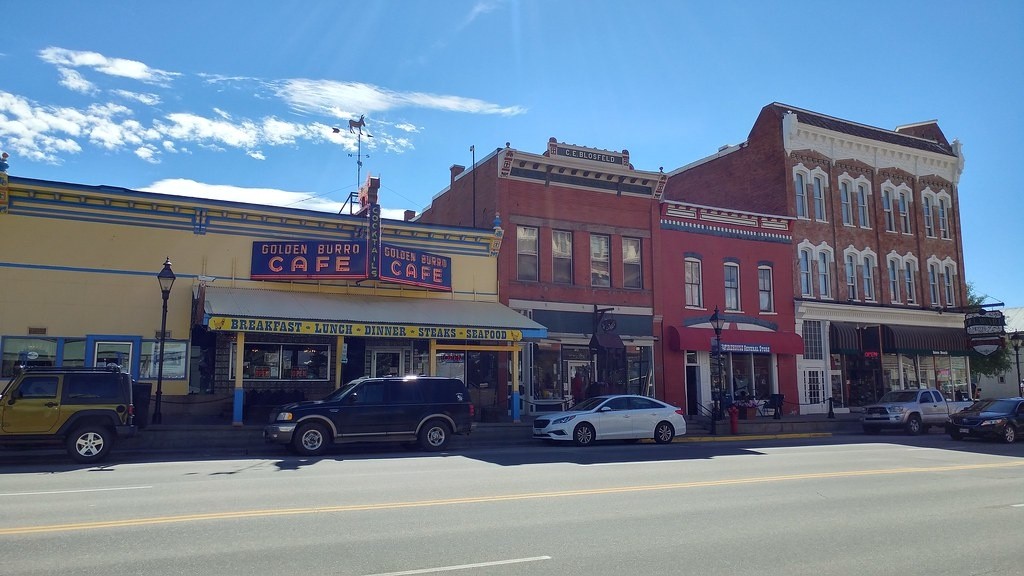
[728,405,740,435]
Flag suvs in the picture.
[0,362,137,464]
[262,374,475,456]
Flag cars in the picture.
[945,397,1024,444]
[532,394,687,446]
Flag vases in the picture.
[738,407,758,420]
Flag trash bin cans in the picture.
[132,383,152,430]
[770,393,785,419]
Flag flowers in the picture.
[732,399,760,408]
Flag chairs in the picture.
[759,393,785,417]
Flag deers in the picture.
[348,114,365,135]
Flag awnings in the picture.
[192,285,548,340]
[830,322,971,357]
[669,327,804,355]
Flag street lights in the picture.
[152,256,177,426]
[1010,329,1023,397]
[709,305,726,420]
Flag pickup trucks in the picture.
[860,388,974,436]
